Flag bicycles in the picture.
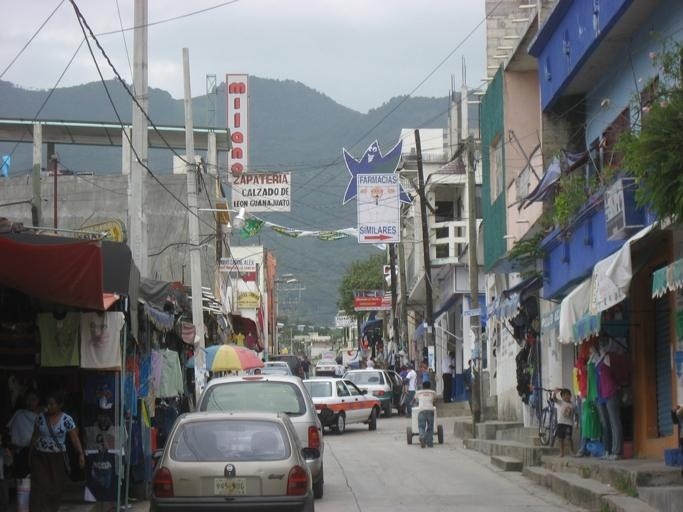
[533,386,559,447]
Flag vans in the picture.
[198,375,325,500]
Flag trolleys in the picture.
[407,402,444,445]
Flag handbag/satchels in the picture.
[62,453,72,475]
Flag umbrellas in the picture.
[184,344,265,371]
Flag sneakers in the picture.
[419,439,432,448]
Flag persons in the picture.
[82,408,131,511]
[441,351,456,402]
[365,341,431,419]
[7,391,40,477]
[297,354,316,380]
[206,371,213,383]
[21,391,86,511]
[461,360,472,415]
[253,368,261,375]
[553,387,579,457]
[578,336,628,461]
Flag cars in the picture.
[250,356,305,378]
[344,370,406,417]
[302,378,381,432]
[315,360,337,375]
[148,410,314,512]
